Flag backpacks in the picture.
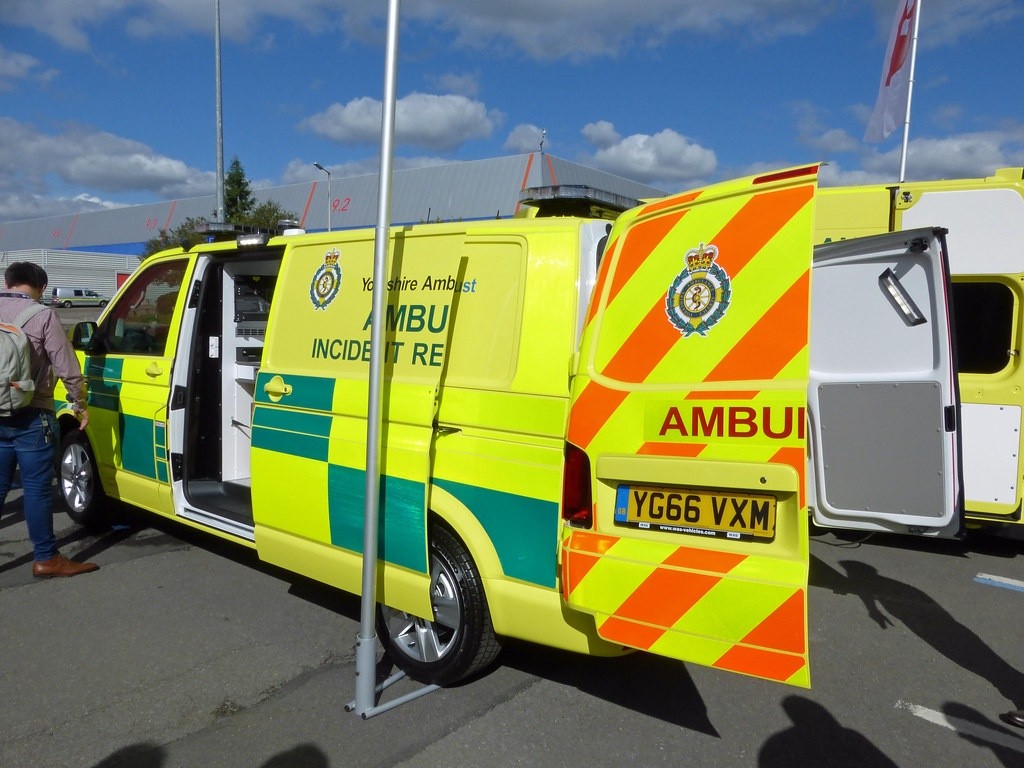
[0,303,53,417]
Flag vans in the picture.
[53,286,111,308]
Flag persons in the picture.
[0,261,98,577]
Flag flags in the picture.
[862,0,919,143]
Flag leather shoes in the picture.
[32,553,98,579]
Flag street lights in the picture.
[314,162,331,233]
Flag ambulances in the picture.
[51,163,1024,691]
